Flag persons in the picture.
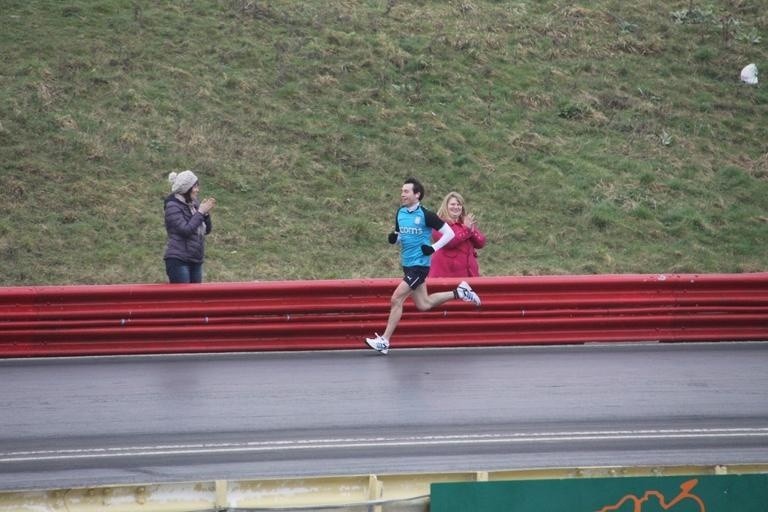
[428,190,487,279]
[161,168,216,285]
[363,177,482,357]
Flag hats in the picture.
[168,169,198,193]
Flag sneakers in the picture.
[364,331,390,355]
[456,281,481,307]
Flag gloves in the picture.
[388,232,398,244]
[421,244,434,256]
